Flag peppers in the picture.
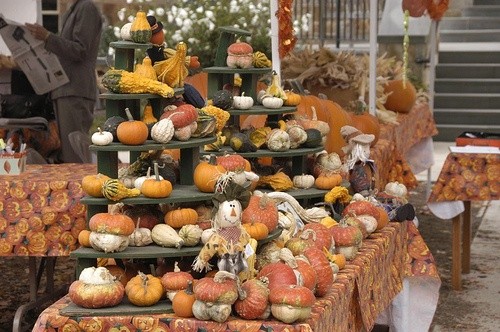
[275,0,297,58]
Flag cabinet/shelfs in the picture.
[59,41,283,316]
[203,27,351,200]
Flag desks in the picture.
[426,152,499,289]
[0,164,179,332]
[369,101,438,194]
[31,220,442,332]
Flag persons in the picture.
[24,0,102,163]
[147,16,205,108]
[342,146,414,222]
[193,177,255,281]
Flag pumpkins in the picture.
[69,10,416,324]
